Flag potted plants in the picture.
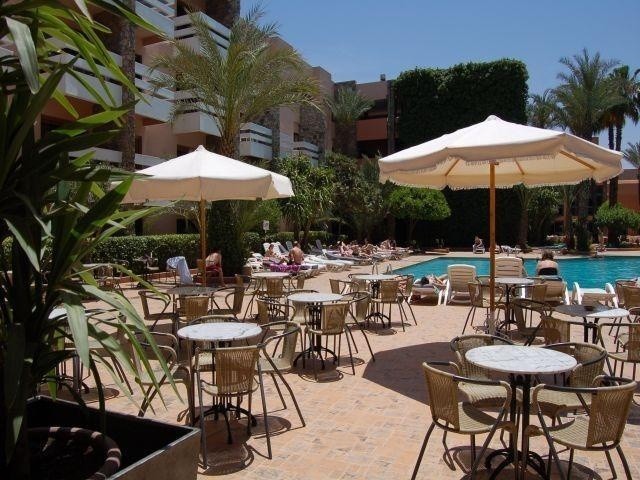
[0,1,203,480]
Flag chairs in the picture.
[90,255,220,288]
[116,272,422,462]
[242,241,414,279]
[6,239,640,480]
[406,256,639,480]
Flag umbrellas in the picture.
[377,115,622,328]
[111,145,295,293]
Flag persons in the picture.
[266,243,274,256]
[290,241,304,265]
[474,235,482,247]
[334,237,397,257]
[205,247,226,288]
[419,276,443,286]
[535,250,558,273]
